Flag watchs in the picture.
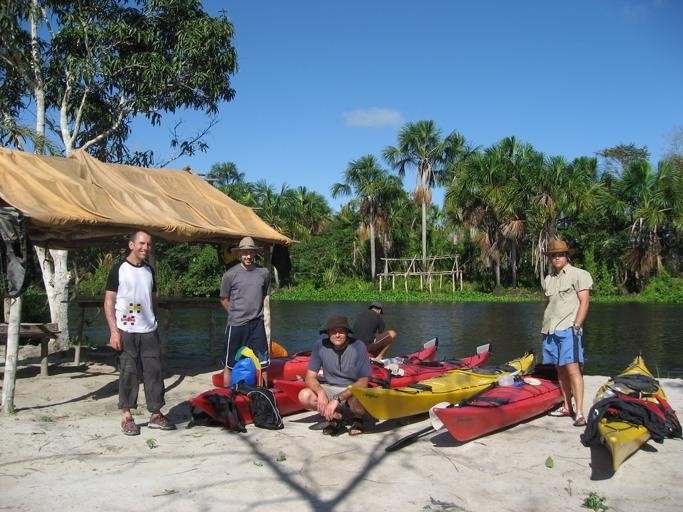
[333,395,342,403]
[573,324,580,331]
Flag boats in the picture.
[189,336,440,428]
[591,352,667,474]
[433,363,565,443]
[347,351,536,421]
[211,350,313,388]
[272,343,493,410]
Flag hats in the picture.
[230,237,264,257]
[541,240,570,256]
[320,316,353,336]
[368,301,384,314]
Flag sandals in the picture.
[322,420,343,435]
[349,417,363,435]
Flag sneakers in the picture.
[548,407,573,417]
[120,417,140,436]
[575,415,586,426]
[147,414,175,430]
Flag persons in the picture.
[351,301,397,360]
[219,237,270,388]
[298,315,371,436]
[104,230,175,436]
[541,241,594,426]
